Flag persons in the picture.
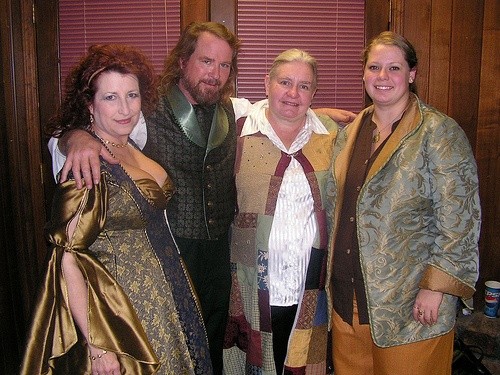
[45,20,366,375]
[326,30,481,375]
[17,46,214,375]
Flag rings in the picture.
[418,309,424,316]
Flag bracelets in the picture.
[88,350,107,360]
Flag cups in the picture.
[484,281,500,319]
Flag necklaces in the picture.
[373,108,405,145]
[95,131,128,149]
